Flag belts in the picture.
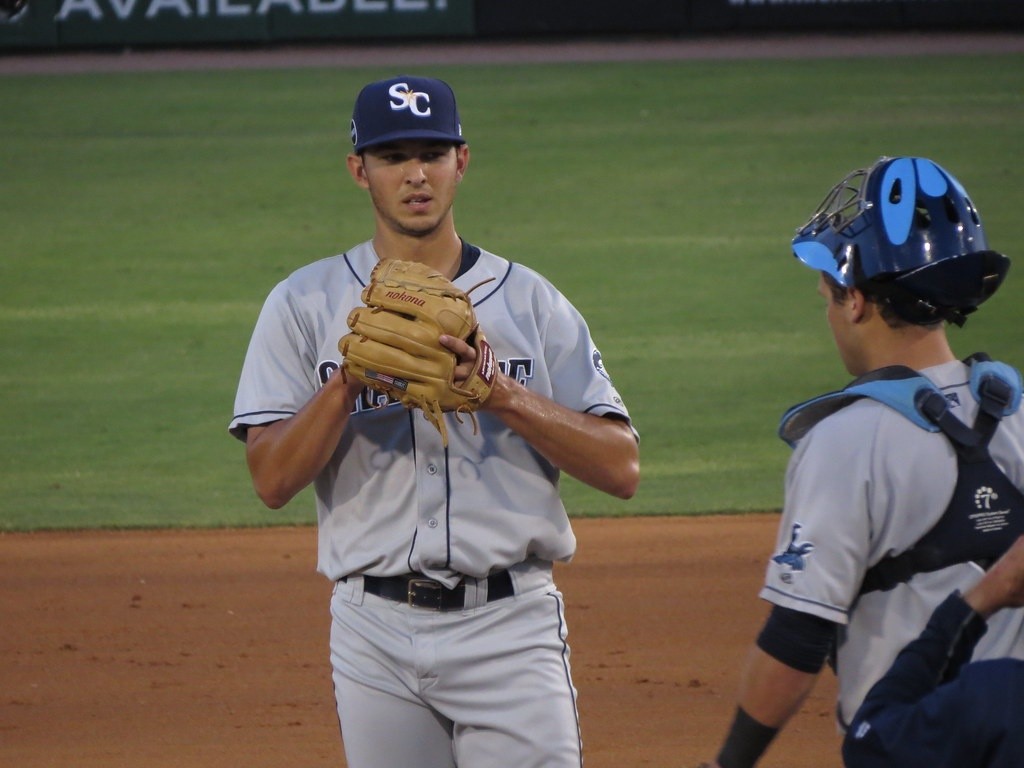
[362,571,512,612]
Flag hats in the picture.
[350,75,467,154]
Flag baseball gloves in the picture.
[336,255,498,415]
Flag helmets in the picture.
[787,156,1013,328]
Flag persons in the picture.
[698,156,1024,767]
[227,76,640,767]
